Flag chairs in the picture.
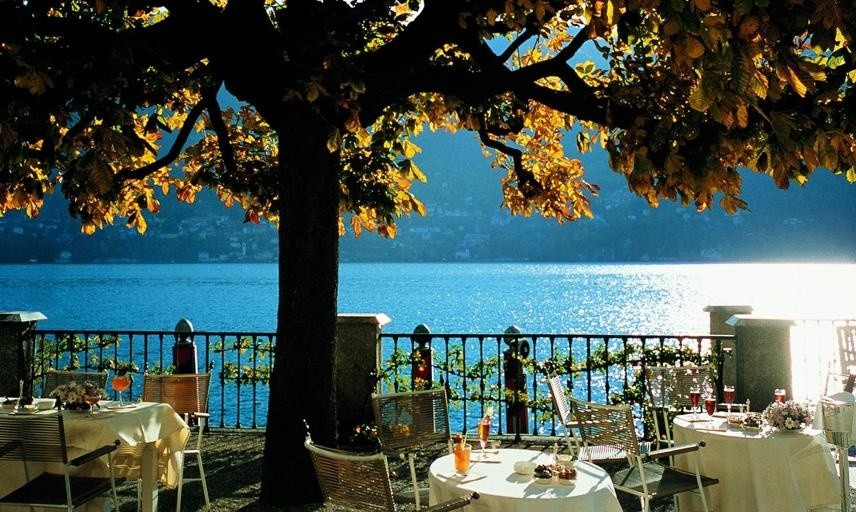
[372,386,454,511]
[569,396,719,512]
[137,360,211,511]
[0,394,126,511]
[546,370,634,467]
[43,360,112,400]
[644,367,718,512]
[303,418,480,511]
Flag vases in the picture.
[64,400,90,410]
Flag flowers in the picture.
[762,400,816,432]
[49,379,110,405]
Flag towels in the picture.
[814,387,856,449]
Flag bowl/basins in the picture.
[37,399,56,409]
[2,405,15,411]
[514,462,536,475]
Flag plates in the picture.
[533,476,552,485]
[557,478,577,486]
[105,402,137,409]
[471,450,502,462]
[0,409,14,414]
[677,414,712,422]
[9,409,39,414]
[714,411,745,418]
[693,422,728,432]
[437,471,487,483]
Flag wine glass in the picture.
[477,418,490,456]
[689,388,700,418]
[705,396,716,423]
[552,439,579,489]
[723,385,735,422]
[774,389,785,404]
[84,389,103,420]
[112,375,130,407]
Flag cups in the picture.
[454,444,472,477]
[448,440,465,453]
